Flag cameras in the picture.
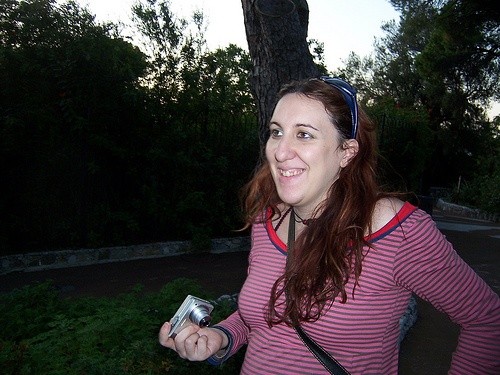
[168,295,214,339]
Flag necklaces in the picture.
[274,206,320,232]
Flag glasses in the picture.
[316,76,359,139]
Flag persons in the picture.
[159,76,500,375]
